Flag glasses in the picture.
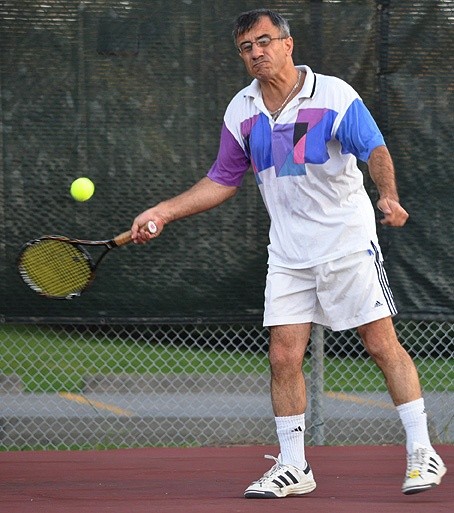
[240,36,287,52]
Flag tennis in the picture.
[70,179,94,202]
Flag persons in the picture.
[131,8,447,499]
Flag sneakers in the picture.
[400,441,448,495]
[243,452,317,499]
